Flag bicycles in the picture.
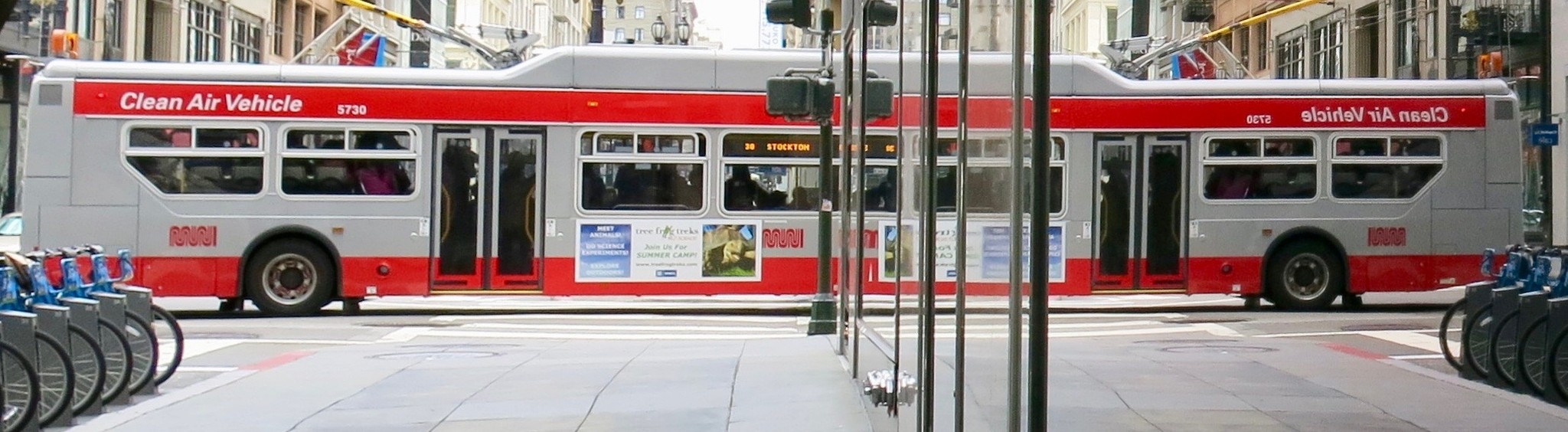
[1516,247,1568,394]
[1439,243,1548,386]
[1547,325,1568,404]
[0,243,185,432]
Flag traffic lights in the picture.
[766,0,811,28]
[866,0,898,28]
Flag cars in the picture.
[1521,209,1544,232]
[0,212,23,257]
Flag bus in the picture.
[13,43,1531,319]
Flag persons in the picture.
[437,140,533,276]
[770,190,790,210]
[352,133,410,195]
[787,187,811,210]
[1100,158,1130,275]
[724,165,775,210]
